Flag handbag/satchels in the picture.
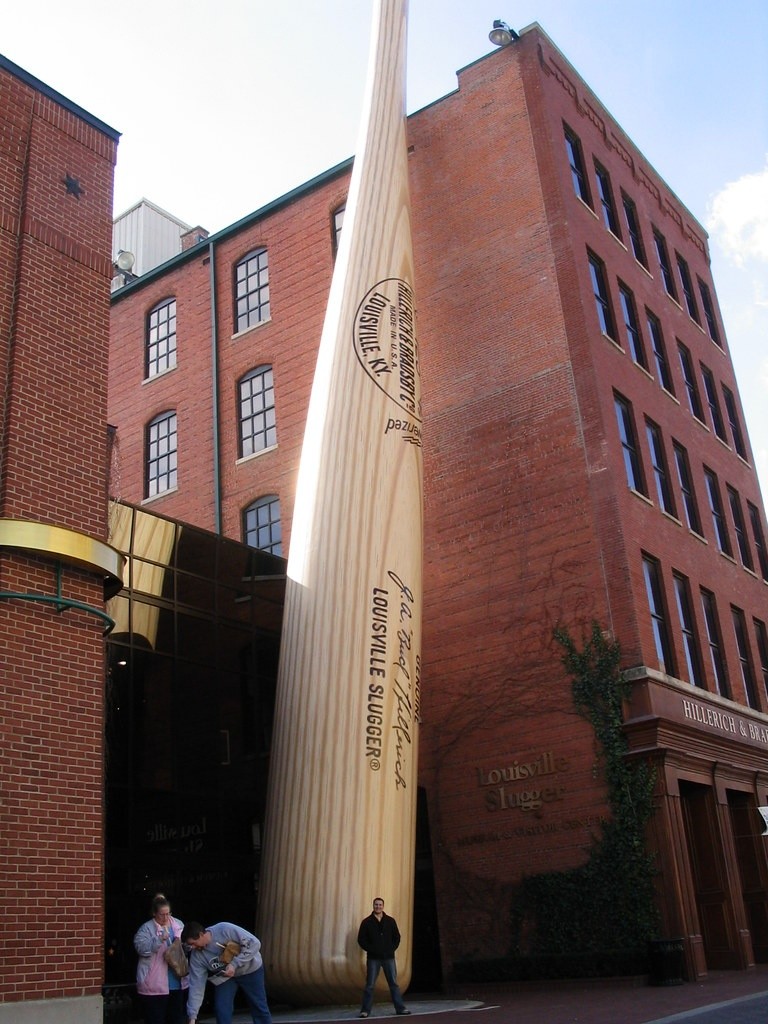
[163,937,192,978]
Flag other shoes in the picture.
[401,1009,411,1014]
[360,1011,368,1017]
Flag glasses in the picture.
[155,913,173,919]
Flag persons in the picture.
[181,921,272,1024]
[133,893,190,1024]
[357,898,412,1018]
